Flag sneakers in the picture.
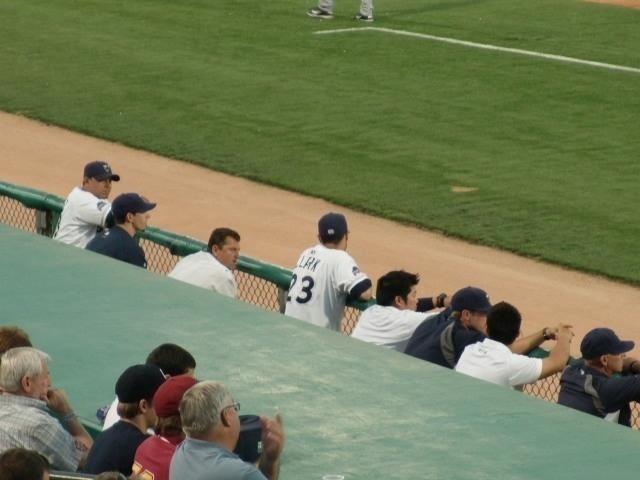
[305,6,335,20]
[350,12,375,23]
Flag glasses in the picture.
[224,399,242,415]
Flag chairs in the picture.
[0,389,140,480]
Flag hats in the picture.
[110,191,158,218]
[148,375,202,419]
[317,211,348,238]
[450,285,495,313]
[114,363,166,404]
[83,160,122,183]
[579,326,636,357]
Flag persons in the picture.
[2,325,285,480]
[558,328,639,428]
[284,212,372,332]
[53,162,120,250]
[349,270,575,391]
[306,0,374,22]
[85,193,156,268]
[168,227,240,297]
